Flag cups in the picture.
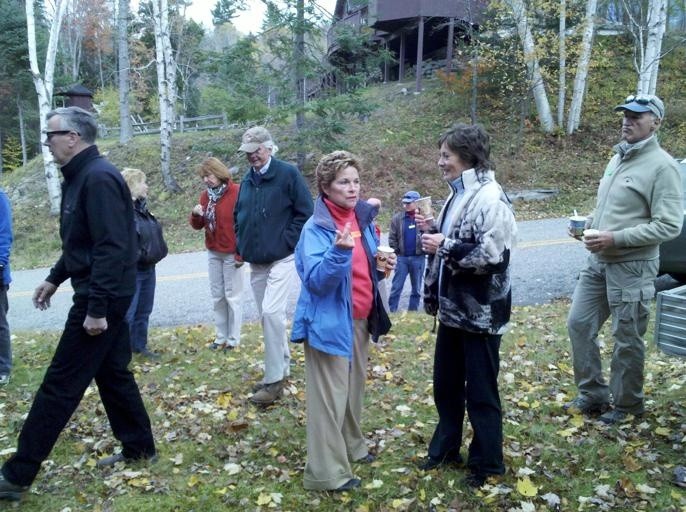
[376,246,394,272]
[415,195,434,221]
[570,216,587,236]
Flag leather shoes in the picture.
[339,454,375,489]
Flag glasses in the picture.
[43,128,80,137]
[625,95,662,117]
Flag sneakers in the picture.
[141,350,156,356]
[562,397,634,423]
[211,343,240,353]
[97,453,156,467]
[421,457,504,487]
[253,379,287,403]
[0,473,28,499]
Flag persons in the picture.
[189,156,245,353]
[366,198,390,315]
[415,126,517,488]
[388,191,426,312]
[0,106,156,501]
[562,94,685,425]
[121,166,168,359]
[0,188,14,384]
[233,127,314,405]
[290,149,397,492]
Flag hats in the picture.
[237,127,272,153]
[402,191,420,203]
[614,95,664,119]
